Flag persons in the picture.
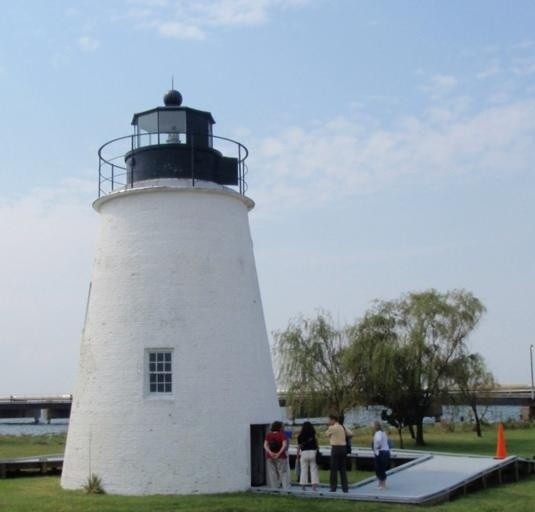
[326,416,352,492]
[298,422,319,490]
[277,425,289,487]
[373,422,388,491]
[262,421,290,489]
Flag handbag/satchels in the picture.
[343,435,354,454]
[316,449,323,465]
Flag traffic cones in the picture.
[495,421,509,460]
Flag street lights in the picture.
[529,344,534,397]
